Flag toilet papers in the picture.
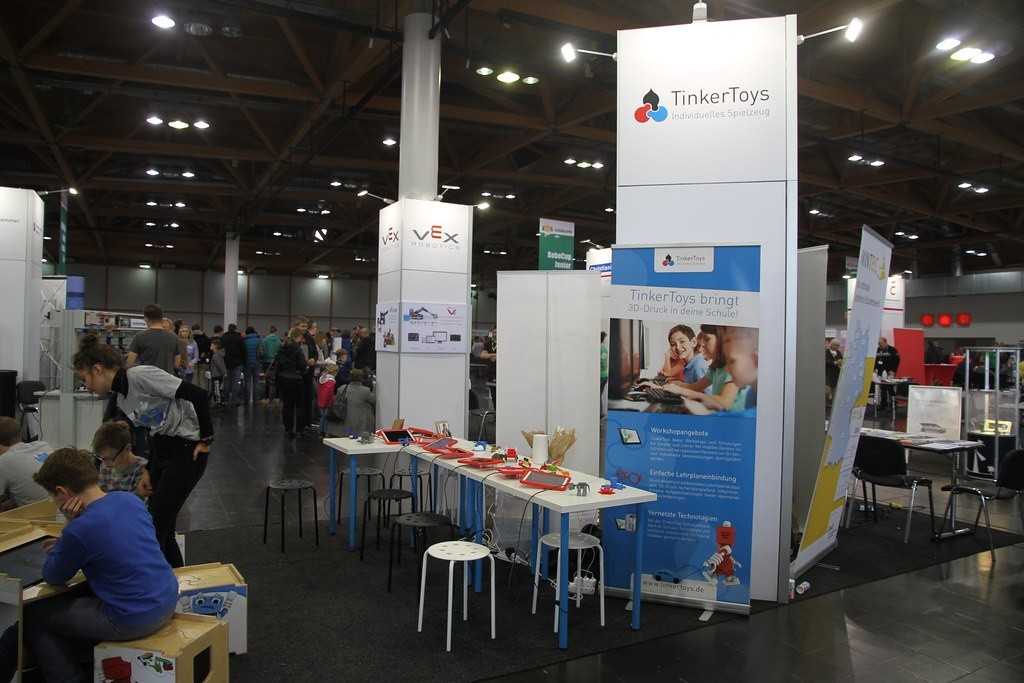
[532,434,548,465]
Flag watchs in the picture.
[199,438,214,446]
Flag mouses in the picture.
[637,378,648,384]
[635,385,650,392]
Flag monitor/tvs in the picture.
[618,428,641,444]
[609,317,650,401]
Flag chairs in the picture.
[845,435,936,544]
[16,381,46,426]
[936,449,1024,560]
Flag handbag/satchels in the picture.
[325,384,348,425]
[264,358,279,380]
[256,338,264,358]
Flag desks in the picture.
[34,390,107,456]
[860,428,984,540]
[0,500,90,683]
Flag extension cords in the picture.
[496,551,521,564]
[567,583,595,595]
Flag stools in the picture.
[359,489,415,584]
[418,541,496,653]
[388,512,453,607]
[531,531,606,633]
[338,466,386,531]
[322,437,657,650]
[164,562,249,655]
[388,468,431,526]
[94,610,230,683]
[264,479,318,553]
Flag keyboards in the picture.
[646,375,684,404]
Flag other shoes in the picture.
[65,673,83,683]
[317,432,329,438]
[295,431,312,439]
[305,426,314,432]
[310,422,321,428]
[260,398,281,405]
[875,402,889,410]
[284,431,298,438]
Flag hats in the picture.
[334,348,348,355]
[228,324,239,330]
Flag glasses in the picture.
[92,444,126,463]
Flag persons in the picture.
[126,304,183,456]
[0,414,65,508]
[469,326,496,425]
[29,446,180,683]
[73,334,214,570]
[162,316,377,443]
[95,420,152,506]
[442,423,451,437]
[657,324,758,416]
[825,338,843,409]
[926,341,1024,425]
[599,331,608,420]
[875,337,900,410]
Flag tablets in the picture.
[376,426,571,490]
[0,535,57,590]
